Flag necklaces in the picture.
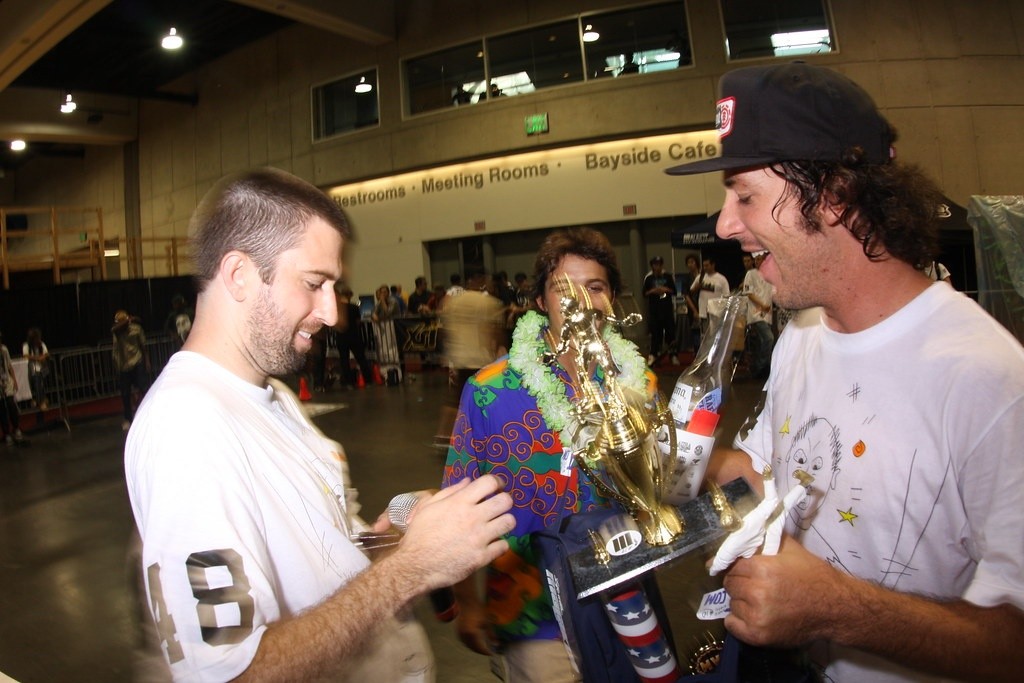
[510,309,647,470]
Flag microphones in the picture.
[389,492,460,624]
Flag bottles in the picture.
[663,294,742,435]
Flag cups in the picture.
[654,424,714,502]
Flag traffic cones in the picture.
[373,364,384,386]
[299,377,313,400]
[355,372,367,390]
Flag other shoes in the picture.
[648,356,655,366]
[672,355,680,366]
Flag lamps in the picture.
[160,25,184,50]
[354,72,372,93]
[583,20,601,43]
[59,78,77,114]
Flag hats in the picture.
[664,59,888,175]
[652,257,663,263]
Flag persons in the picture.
[111,312,150,431]
[301,268,534,438]
[0,344,22,447]
[664,61,1024,683]
[640,251,775,383]
[123,163,518,683]
[23,329,50,410]
[439,227,710,683]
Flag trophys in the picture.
[544,296,683,547]
